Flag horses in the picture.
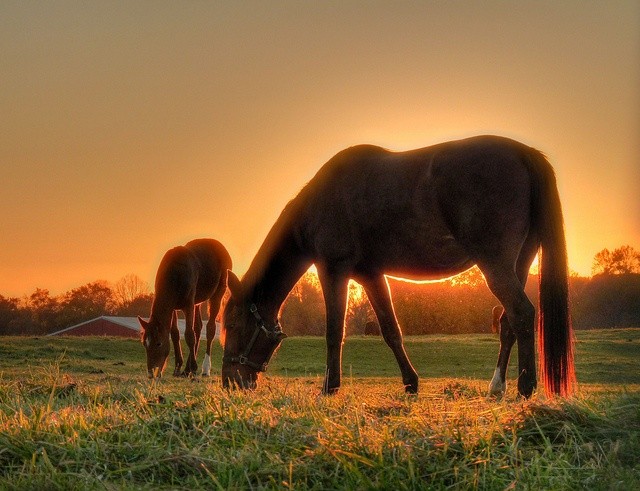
[222,135,580,402]
[137,238,233,382]
